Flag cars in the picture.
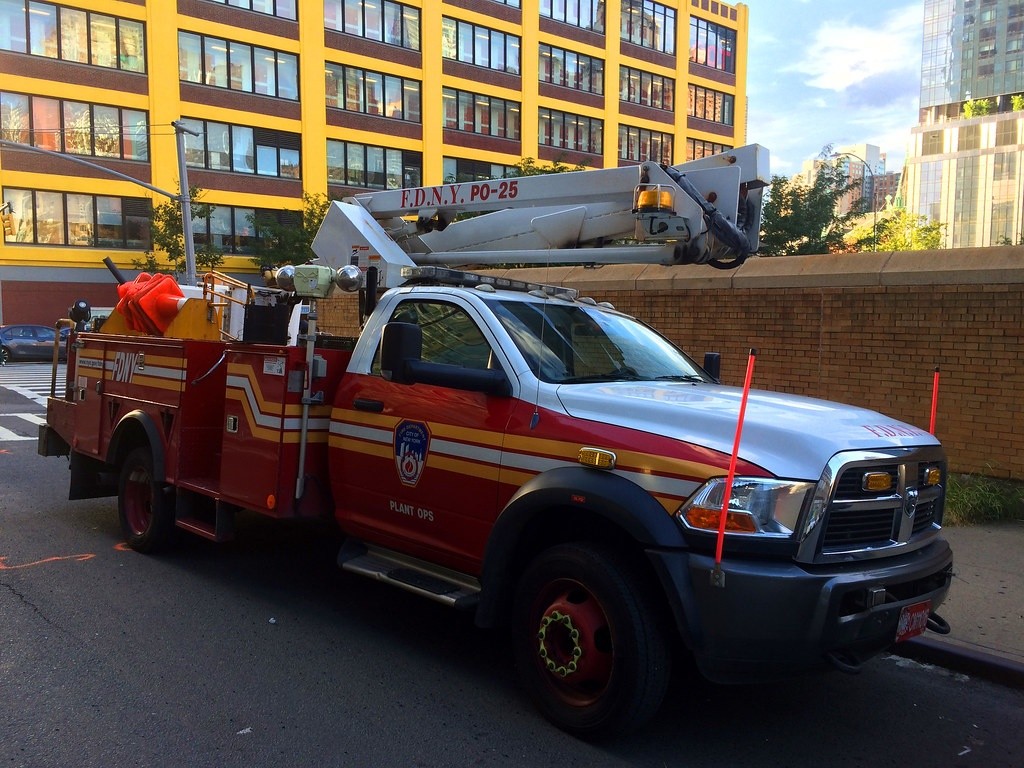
[59,325,92,337]
[0,325,70,363]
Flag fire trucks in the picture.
[33,145,959,738]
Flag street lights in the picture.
[172,118,207,288]
[828,151,879,253]
